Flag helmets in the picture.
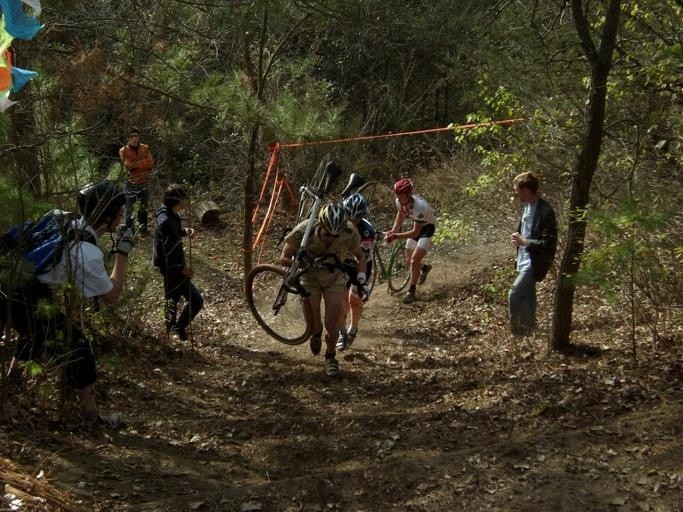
[319,202,349,235]
[394,179,413,195]
[77,181,126,228]
[343,192,368,222]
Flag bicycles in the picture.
[337,171,376,218]
[244,153,344,348]
[350,228,412,301]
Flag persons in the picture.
[384,176,437,304]
[279,201,363,377]
[507,170,559,338]
[118,125,155,234]
[32,177,138,437]
[334,192,378,352]
[150,183,205,342]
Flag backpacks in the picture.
[0,213,97,310]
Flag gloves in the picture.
[114,225,137,258]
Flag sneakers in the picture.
[45,424,73,444]
[324,358,340,378]
[336,332,347,351]
[346,327,358,346]
[170,325,188,341]
[419,264,432,285]
[74,415,120,434]
[403,295,416,303]
[310,323,323,356]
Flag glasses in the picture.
[119,208,124,216]
